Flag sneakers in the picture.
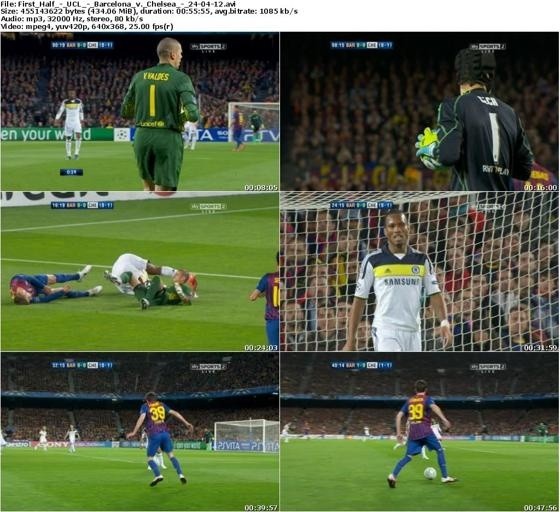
[102,270,122,286]
[387,443,457,488]
[87,285,103,297]
[188,275,198,291]
[140,297,149,311]
[76,264,92,281]
[148,464,187,486]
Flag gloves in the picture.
[415,126,442,160]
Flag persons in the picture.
[249,251,279,351]
[1,52,278,162]
[280,194,559,351]
[1,352,279,486]
[9,264,104,305]
[104,268,193,310]
[280,355,559,459]
[121,37,200,191]
[281,46,559,191]
[112,252,197,295]
[387,379,458,489]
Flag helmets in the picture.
[454,46,497,92]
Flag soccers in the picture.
[422,129,444,170]
[424,467,437,480]
